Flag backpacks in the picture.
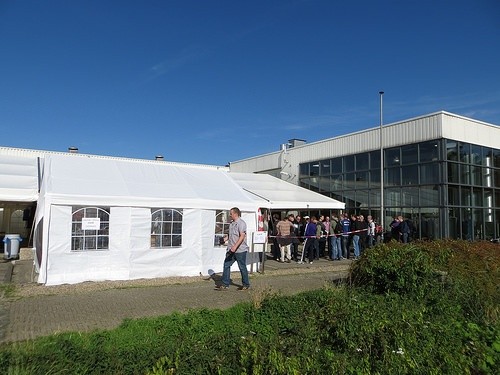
[332,218,344,237]
[374,221,382,236]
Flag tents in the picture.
[30,156,345,286]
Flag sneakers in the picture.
[237,285,250,291]
[214,284,229,290]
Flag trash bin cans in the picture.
[3,234,21,259]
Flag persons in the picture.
[268,213,375,265]
[213,207,251,291]
[389,215,409,243]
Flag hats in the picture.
[303,216,310,218]
